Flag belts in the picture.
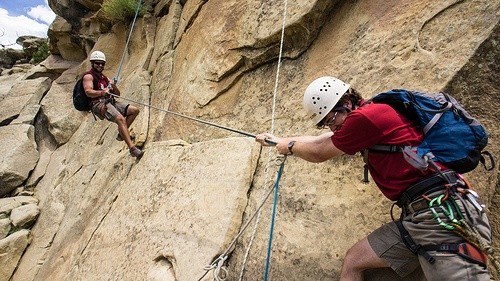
[397,171,459,207]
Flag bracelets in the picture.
[101,90,104,96]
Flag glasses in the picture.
[94,62,105,66]
[323,113,339,130]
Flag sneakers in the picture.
[129,146,144,157]
[116,133,133,141]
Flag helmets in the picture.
[89,51,106,61]
[302,76,350,124]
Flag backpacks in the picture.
[73,76,98,121]
[352,88,496,185]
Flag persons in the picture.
[82,50,145,158]
[255,76,496,281]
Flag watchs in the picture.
[288,140,296,154]
